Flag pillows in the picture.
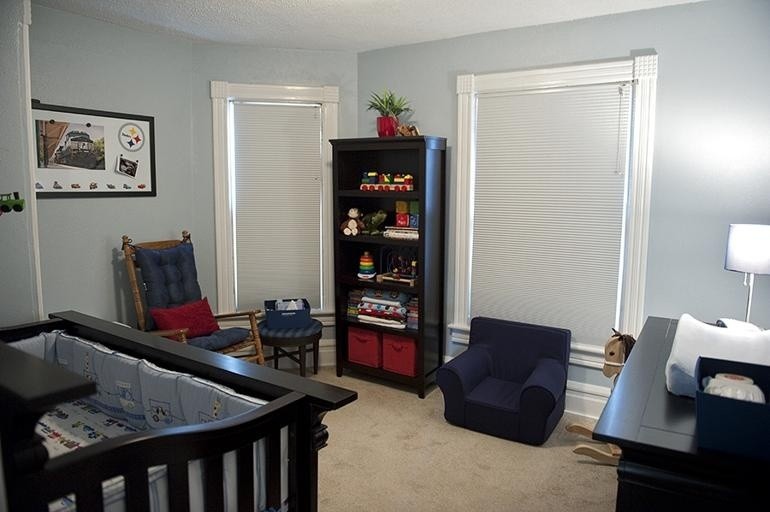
[147,296,221,342]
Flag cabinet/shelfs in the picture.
[327,135,447,399]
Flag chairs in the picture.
[436,314,571,446]
[121,230,265,366]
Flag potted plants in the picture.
[367,86,414,138]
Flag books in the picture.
[405,296,418,330]
[346,289,367,322]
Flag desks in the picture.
[592,315,770,512]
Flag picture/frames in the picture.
[32,98,157,199]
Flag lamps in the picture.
[724,223,770,324]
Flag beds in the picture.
[0,309,358,511]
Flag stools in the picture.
[257,318,323,377]
